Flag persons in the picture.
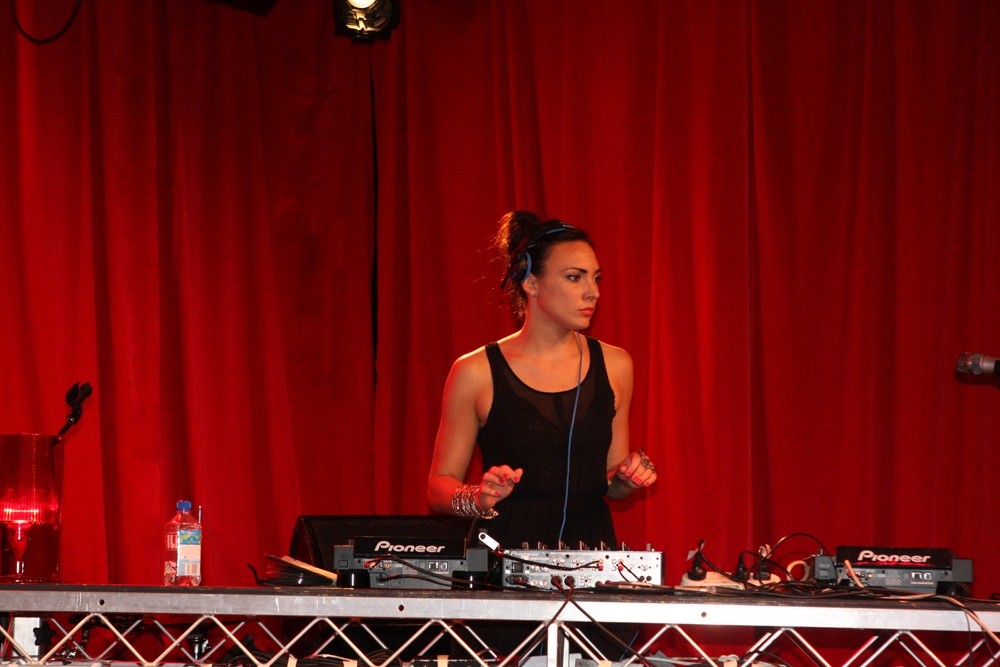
[426,210,658,656]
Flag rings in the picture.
[640,457,655,471]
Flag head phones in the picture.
[508,223,576,284]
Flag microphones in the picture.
[500,274,507,289]
[955,349,1000,377]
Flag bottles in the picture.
[162,500,202,588]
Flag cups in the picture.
[0,435,64,588]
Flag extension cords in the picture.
[680,571,782,590]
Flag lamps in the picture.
[333,0,400,44]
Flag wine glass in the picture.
[4,511,37,583]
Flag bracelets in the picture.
[454,485,499,520]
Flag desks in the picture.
[0,583,1000,667]
